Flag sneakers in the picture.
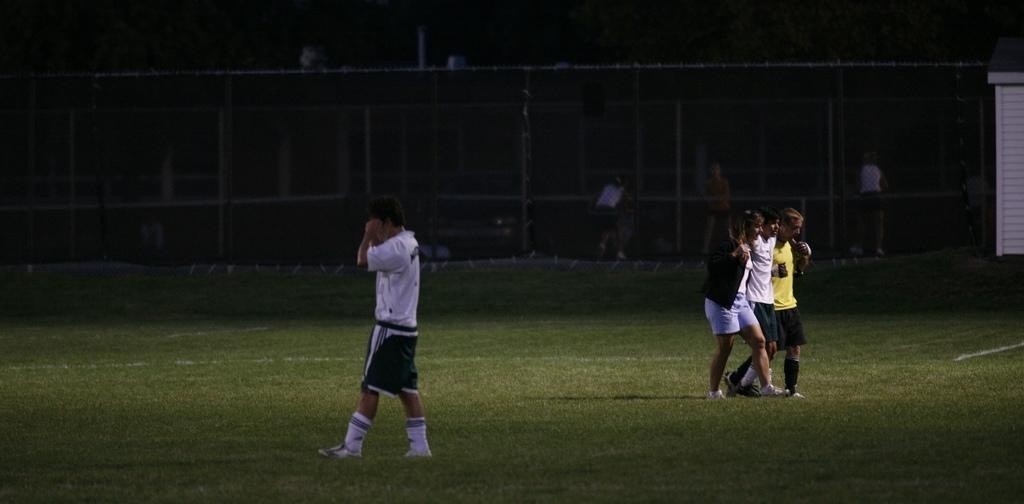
[317,443,362,460]
[707,369,805,401]
[402,450,432,458]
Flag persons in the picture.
[317,194,432,459]
[704,208,811,399]
[702,163,731,255]
[849,151,888,257]
[592,177,633,260]
